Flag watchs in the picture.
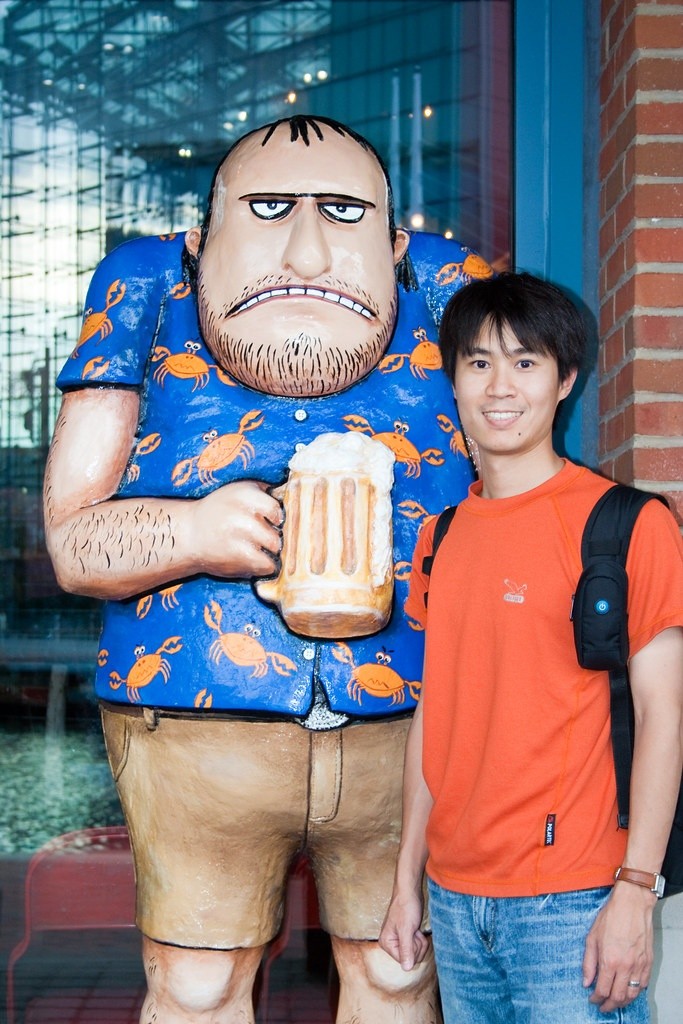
[614,866,665,899]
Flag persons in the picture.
[42,114,491,1023]
[378,269,683,1024]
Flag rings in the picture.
[628,982,640,987]
